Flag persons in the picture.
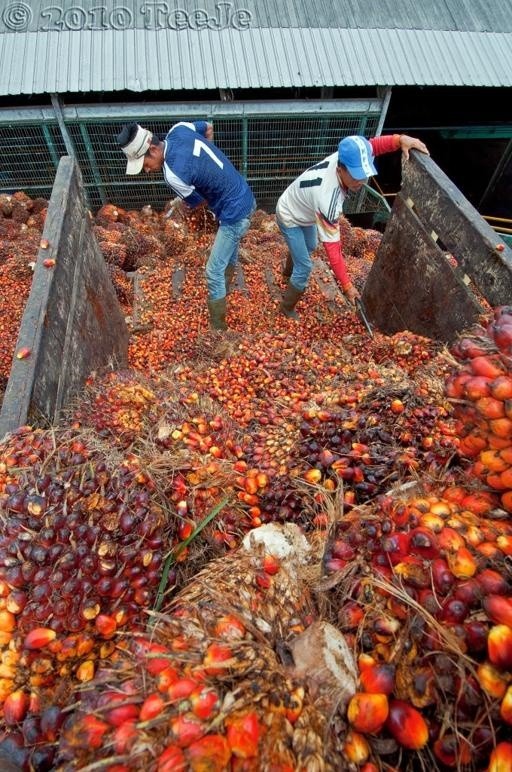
[118,120,256,334]
[275,134,428,322]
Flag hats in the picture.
[338,135,378,180]
[118,124,153,176]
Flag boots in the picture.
[282,251,293,276]
[280,279,305,320]
[225,263,234,294]
[206,295,227,330]
